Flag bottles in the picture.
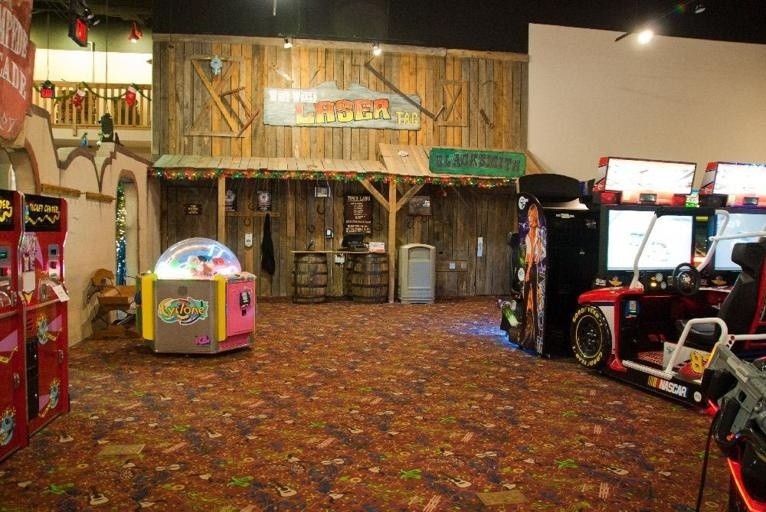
[498,298,519,327]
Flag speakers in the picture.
[705,210,766,277]
[598,205,697,276]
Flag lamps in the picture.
[90,18,101,25]
[80,1,94,20]
[284,37,382,56]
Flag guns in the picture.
[715,345,766,435]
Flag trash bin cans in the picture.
[398,242,436,304]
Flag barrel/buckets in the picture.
[343,255,389,303]
[291,253,328,302]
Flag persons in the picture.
[517,204,543,344]
